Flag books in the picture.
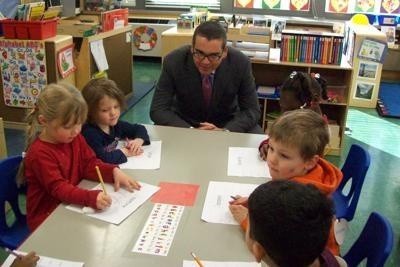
[17,0,63,22]
[101,8,129,32]
[279,34,343,65]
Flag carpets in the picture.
[4,80,155,156]
[377,81,400,117]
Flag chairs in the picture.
[0,155,31,249]
[332,143,370,221]
[342,211,394,265]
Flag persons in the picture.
[150,20,265,134]
[79,77,150,164]
[16,84,141,233]
[0,243,39,267]
[245,177,348,267]
[229,109,344,256]
[259,70,331,164]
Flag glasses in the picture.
[191,45,225,62]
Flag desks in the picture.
[1,123,272,266]
[161,26,271,75]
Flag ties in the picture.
[201,74,213,110]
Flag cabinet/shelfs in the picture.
[248,57,354,156]
[1,33,77,132]
[58,21,133,98]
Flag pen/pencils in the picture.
[5,248,22,260]
[231,196,237,200]
[125,137,130,144]
[95,166,108,195]
[191,252,204,267]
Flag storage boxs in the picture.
[0,18,59,40]
